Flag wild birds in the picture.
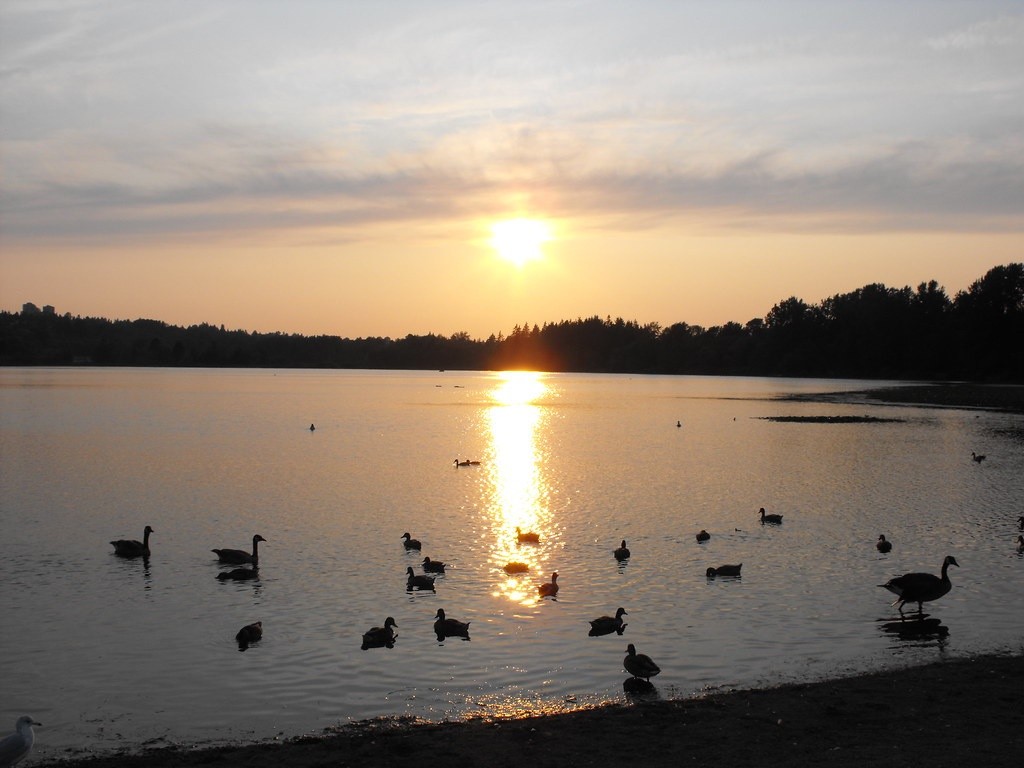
[504,562,529,574]
[623,644,662,682]
[400,532,422,551]
[422,557,446,570]
[361,617,398,644]
[588,607,628,637]
[758,507,783,525]
[453,459,480,466]
[211,534,267,565]
[434,608,470,636]
[676,421,681,427]
[235,621,263,649]
[538,571,559,597]
[516,527,540,541]
[876,534,892,553]
[970,452,986,464]
[310,424,315,431]
[876,556,960,611]
[706,563,743,578]
[0,716,43,768]
[613,540,631,561]
[696,530,711,544]
[406,566,436,589]
[110,525,154,558]
[1015,516,1024,553]
